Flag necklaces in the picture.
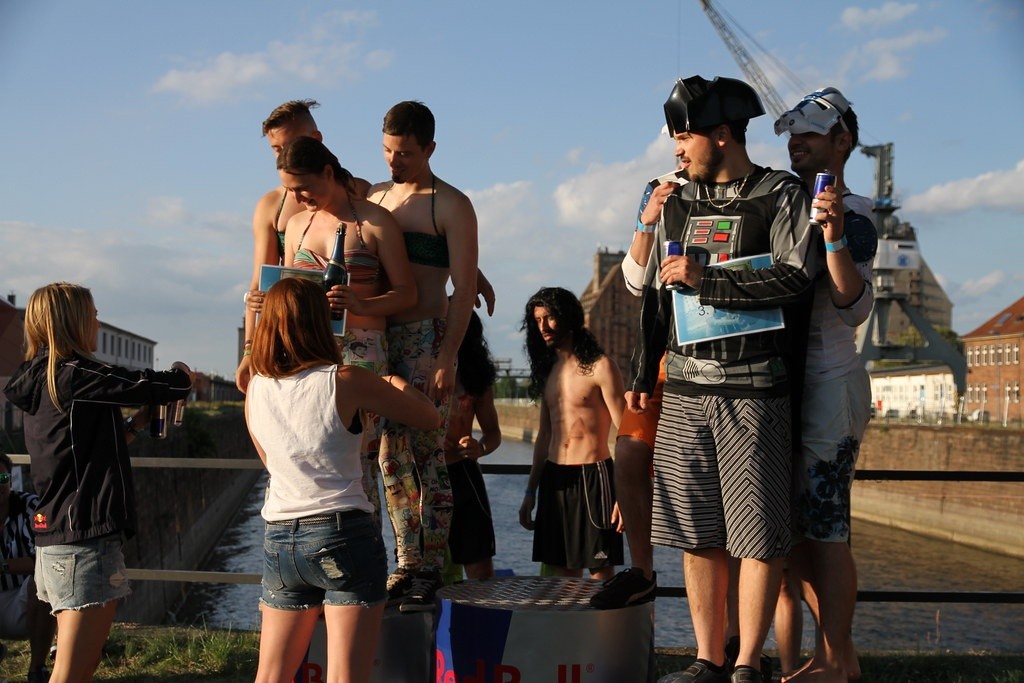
[704,164,754,208]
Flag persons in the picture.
[591,77,878,683]
[9,281,196,683]
[244,277,443,683]
[0,450,57,683]
[518,288,627,579]
[235,98,501,613]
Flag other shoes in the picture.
[658,663,729,683]
[730,668,767,683]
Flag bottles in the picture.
[323,222,348,320]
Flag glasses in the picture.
[0,473,11,484]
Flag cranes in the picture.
[698,0,897,201]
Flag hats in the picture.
[664,76,766,146]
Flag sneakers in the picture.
[385,568,413,607]
[589,566,658,609]
[399,571,442,611]
[726,635,773,683]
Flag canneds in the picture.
[808,169,837,224]
[148,399,184,439]
[662,238,684,290]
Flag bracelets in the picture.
[525,489,536,497]
[637,219,657,234]
[825,234,848,253]
[243,292,248,303]
[244,340,252,356]
[480,441,486,455]
[389,373,396,385]
